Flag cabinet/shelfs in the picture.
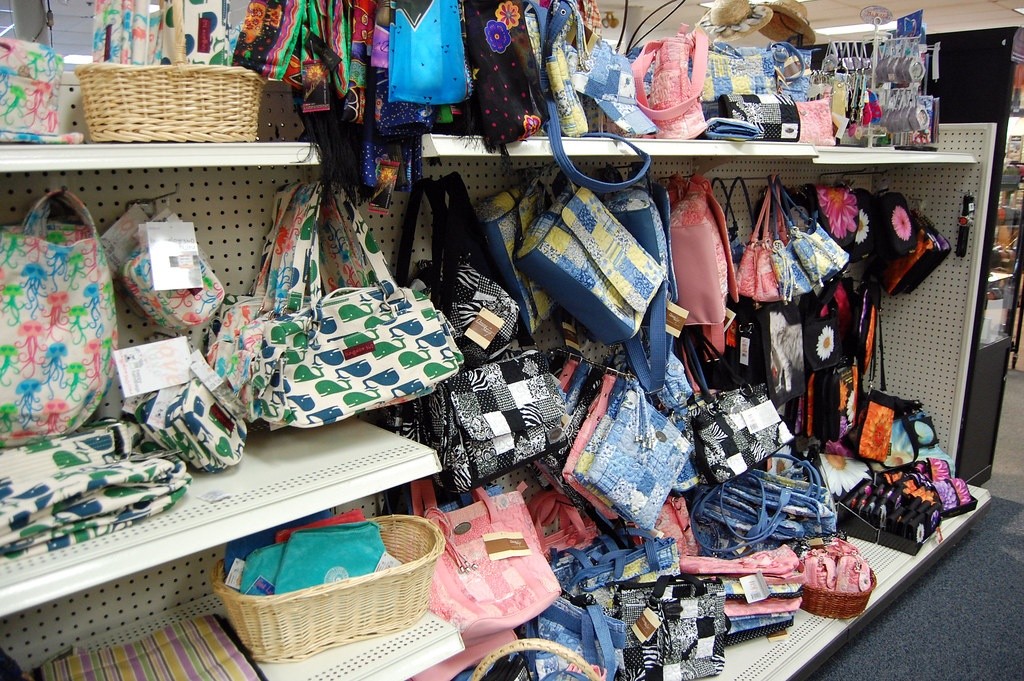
[0,122,996,681]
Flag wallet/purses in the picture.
[705,118,760,139]
[842,457,971,546]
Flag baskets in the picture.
[74,0,265,143]
[209,514,446,664]
[799,568,877,619]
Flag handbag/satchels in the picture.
[632,23,836,147]
[768,170,956,503]
[0,176,465,565]
[231,0,625,193]
[0,38,84,145]
[395,173,837,681]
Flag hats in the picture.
[698,0,815,48]
[570,40,660,135]
